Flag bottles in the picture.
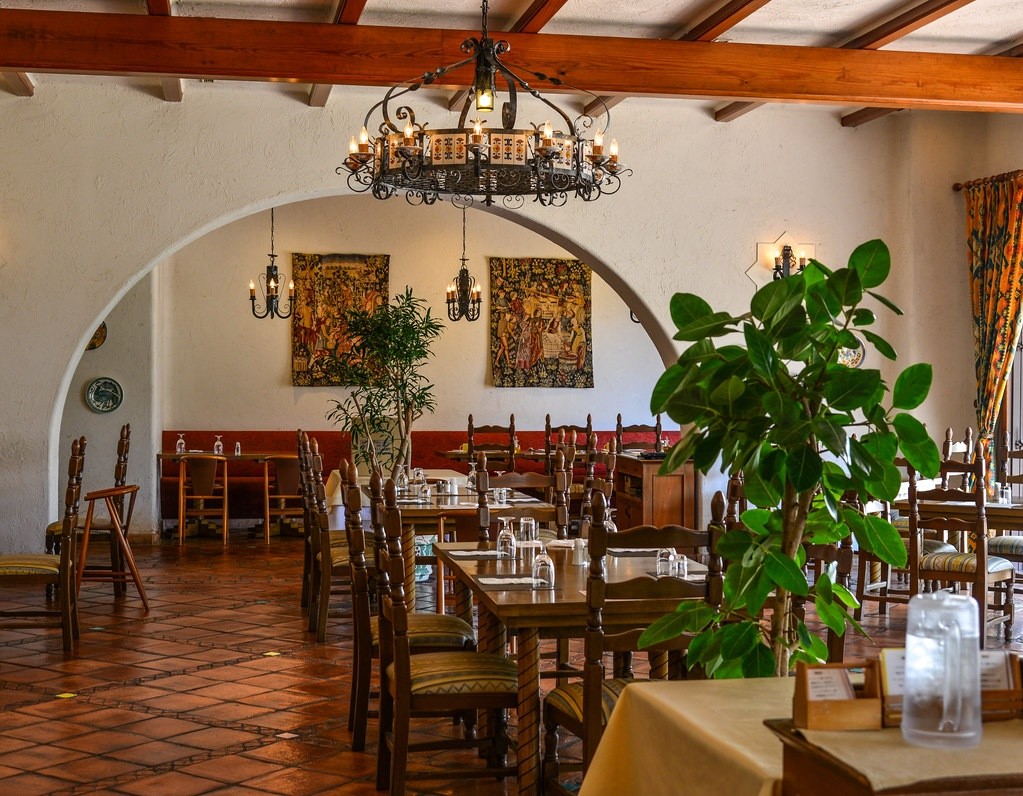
[234,442,240,456]
[581,517,591,538]
[991,482,1012,505]
[513,439,520,452]
[660,436,670,453]
[435,477,458,495]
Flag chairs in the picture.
[0,413,1023,796]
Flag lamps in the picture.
[445,207,482,323]
[333,0,634,209]
[247,207,295,319]
[771,245,807,281]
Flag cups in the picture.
[668,554,688,580]
[413,468,424,484]
[899,590,983,748]
[519,517,536,541]
[603,442,609,450]
[494,488,506,506]
[533,564,551,588]
[499,534,512,557]
[656,546,678,578]
[459,443,468,454]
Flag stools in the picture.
[76,484,152,611]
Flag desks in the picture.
[434,543,776,796]
[889,494,1023,595]
[764,719,1023,796]
[360,484,560,541]
[581,676,798,796]
[325,469,469,505]
[434,448,608,473]
[158,451,324,535]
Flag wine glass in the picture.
[496,516,517,560]
[467,462,478,488]
[418,473,431,503]
[214,434,224,454]
[176,434,185,452]
[603,507,618,534]
[532,538,556,590]
[493,470,507,506]
[396,464,410,491]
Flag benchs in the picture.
[160,430,681,540]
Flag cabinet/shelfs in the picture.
[616,453,695,557]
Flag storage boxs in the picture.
[980,651,1023,722]
[791,656,883,732]
